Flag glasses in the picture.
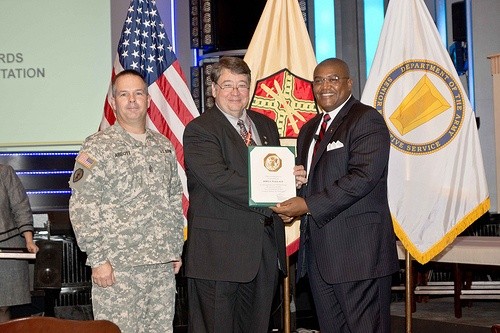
[312,75,349,86]
[216,82,250,93]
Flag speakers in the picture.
[34,239,64,291]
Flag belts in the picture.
[264,216,273,227]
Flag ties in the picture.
[311,114,331,166]
[237,119,257,147]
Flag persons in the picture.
[182,57,309,333]
[0,162,39,324]
[68,69,184,333]
[268,58,400,333]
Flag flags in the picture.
[98,0,201,242]
[360,0,492,266]
[242,0,324,257]
